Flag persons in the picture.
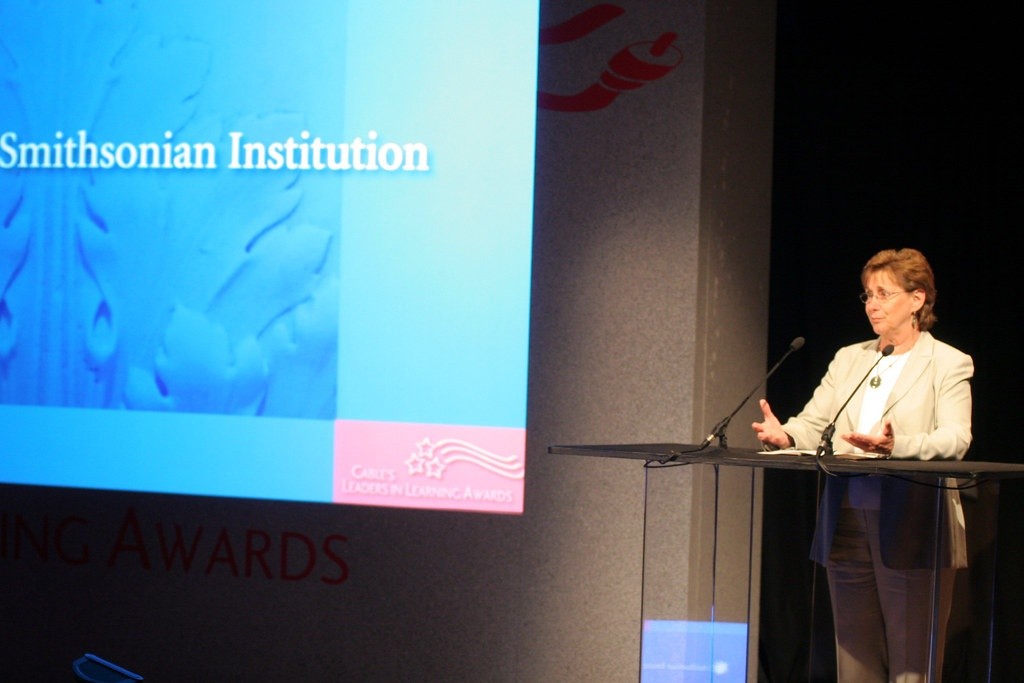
[752,248,973,683]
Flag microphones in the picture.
[817,344,894,454]
[699,336,805,451]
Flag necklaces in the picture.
[870,348,911,388]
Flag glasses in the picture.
[860,290,906,304]
